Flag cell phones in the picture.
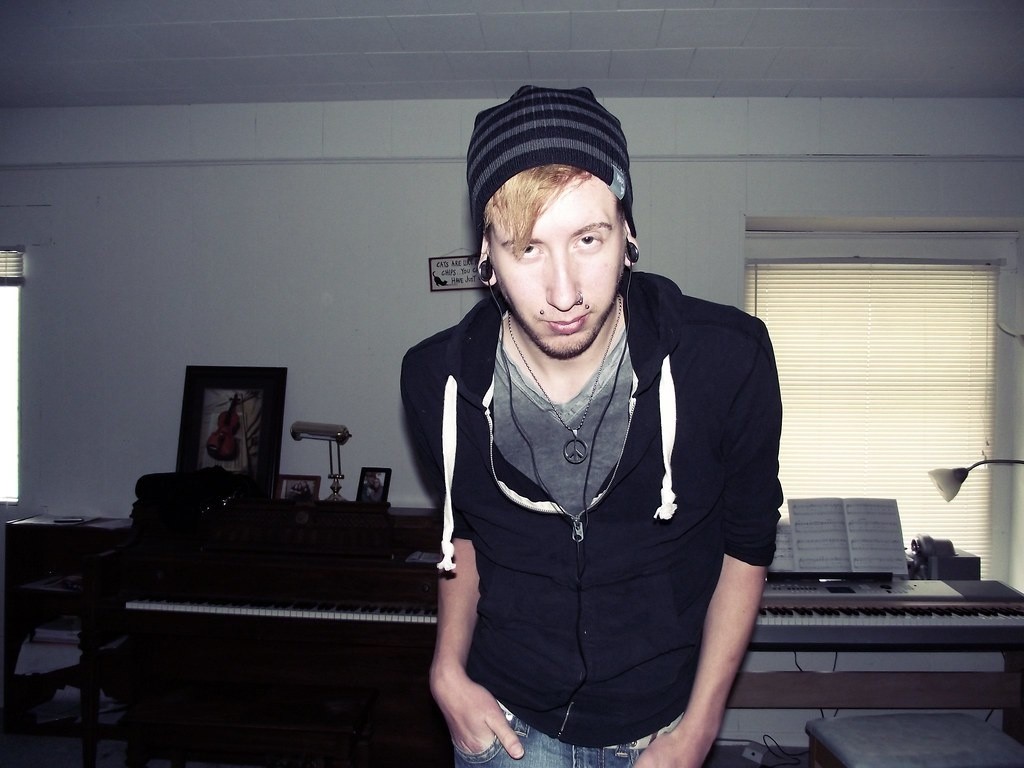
[53,518,84,523]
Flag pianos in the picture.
[722,570,1024,645]
[71,493,454,768]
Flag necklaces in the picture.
[508,291,622,463]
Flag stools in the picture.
[804,714,1024,768]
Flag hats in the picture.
[466,85,636,248]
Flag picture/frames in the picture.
[175,365,288,509]
[275,475,322,501]
[356,466,392,504]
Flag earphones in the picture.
[625,238,639,263]
[480,255,492,282]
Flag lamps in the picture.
[932,459,1024,502]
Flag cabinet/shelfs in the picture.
[0,515,134,768]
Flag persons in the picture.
[289,481,311,498]
[359,471,377,500]
[399,85,784,768]
[368,478,383,501]
